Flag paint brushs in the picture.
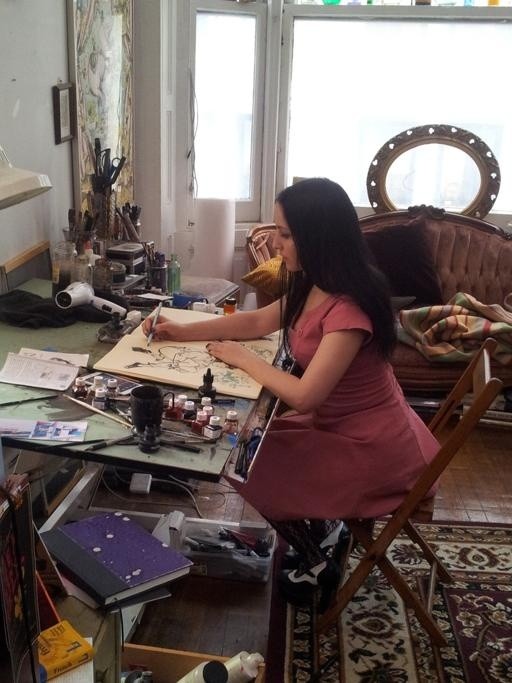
[68,191,101,256]
[114,202,142,243]
[34,438,113,450]
[118,438,217,446]
[62,394,134,429]
[0,395,59,406]
[161,430,211,441]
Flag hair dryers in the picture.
[55,281,127,319]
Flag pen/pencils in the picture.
[187,398,235,404]
[89,138,126,184]
[147,302,163,346]
[235,363,290,480]
[160,439,200,453]
[84,433,134,454]
[141,241,166,293]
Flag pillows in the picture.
[241,254,292,299]
[362,213,443,314]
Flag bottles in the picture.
[52,241,112,299]
[168,253,181,293]
[73,376,119,410]
[223,297,238,317]
[166,394,238,440]
[175,650,264,683]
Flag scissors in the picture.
[102,154,120,180]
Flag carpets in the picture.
[264,515,512,682]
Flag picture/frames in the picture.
[51,81,77,145]
[1,482,41,682]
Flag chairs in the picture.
[314,336,503,647]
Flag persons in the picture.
[141,177,444,612]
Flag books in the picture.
[0,346,90,391]
[110,588,172,615]
[38,619,96,682]
[40,509,194,607]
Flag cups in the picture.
[129,383,175,429]
[147,266,166,293]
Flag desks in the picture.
[0,275,285,681]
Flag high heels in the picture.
[277,521,353,614]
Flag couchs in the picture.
[244,203,510,392]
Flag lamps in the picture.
[1,147,52,210]
